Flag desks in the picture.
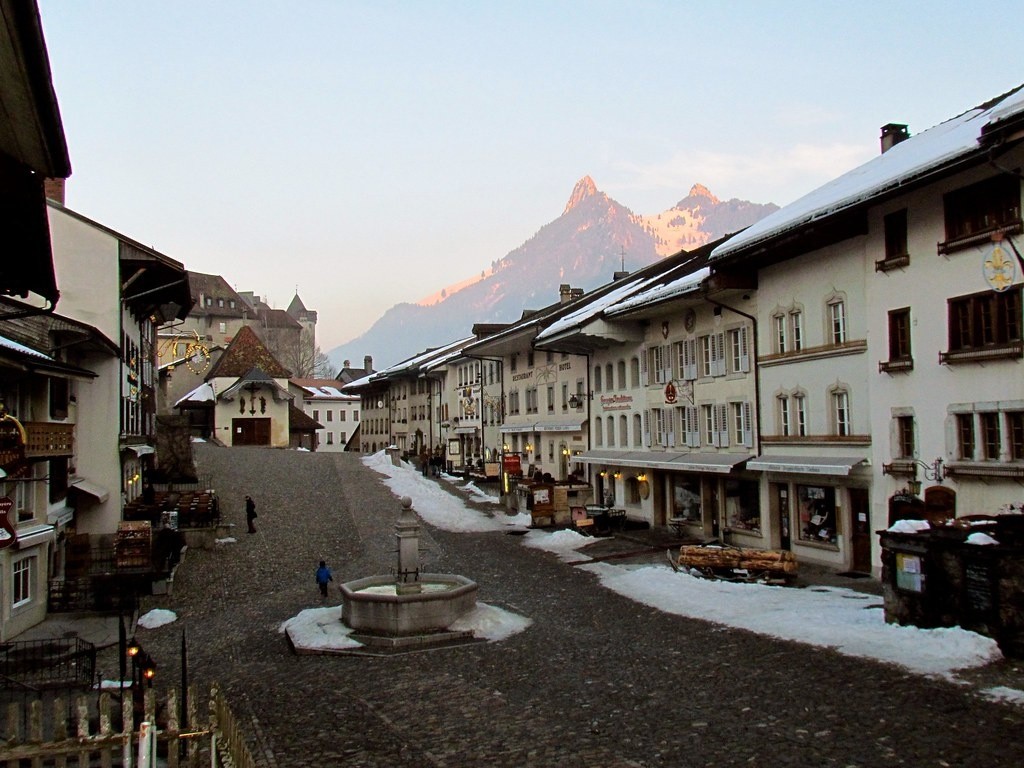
[669,517,687,537]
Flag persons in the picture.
[421,443,445,480]
[245,495,258,534]
[464,458,482,482]
[316,560,333,598]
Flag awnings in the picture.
[451,427,480,435]
[604,265,714,318]
[709,107,986,260]
[535,277,646,354]
[747,455,869,475]
[67,478,110,505]
[501,418,586,433]
[173,383,214,409]
[120,445,155,457]
[988,87,1024,148]
[569,450,755,474]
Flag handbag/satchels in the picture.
[248,511,258,520]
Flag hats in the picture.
[319,560,325,565]
[245,495,249,498]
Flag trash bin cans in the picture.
[447,460,454,470]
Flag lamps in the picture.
[563,446,571,459]
[907,457,943,495]
[614,471,621,478]
[568,390,594,408]
[527,442,533,454]
[600,469,607,476]
[638,472,646,481]
[504,443,510,453]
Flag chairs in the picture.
[123,488,218,527]
